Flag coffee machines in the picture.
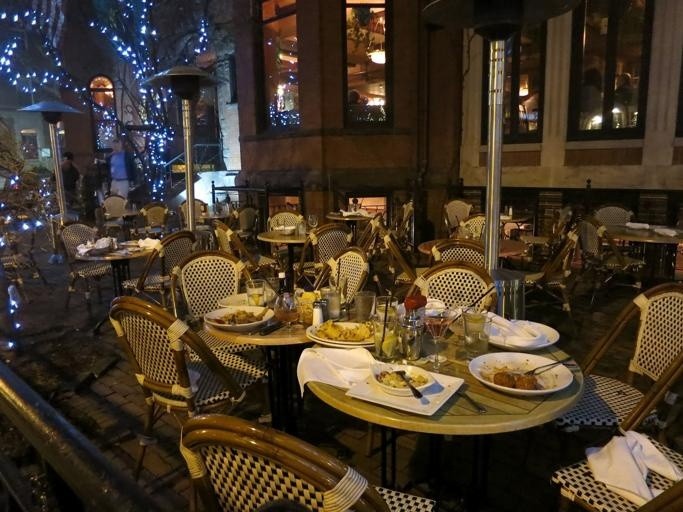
[376,295,398,324]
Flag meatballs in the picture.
[516,377,539,390]
[494,372,516,388]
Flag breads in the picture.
[379,369,425,391]
[320,321,378,345]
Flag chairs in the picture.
[0,211,49,304]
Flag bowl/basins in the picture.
[367,362,436,396]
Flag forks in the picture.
[392,370,422,400]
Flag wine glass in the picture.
[308,215,319,229]
[274,293,301,335]
[416,307,458,375]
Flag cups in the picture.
[320,286,341,321]
[461,306,488,357]
[373,321,401,363]
[294,221,307,241]
[355,291,375,322]
[246,279,265,306]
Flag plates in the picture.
[345,363,464,416]
[468,352,575,397]
[306,321,388,349]
[478,320,560,353]
[203,306,274,332]
[218,290,279,307]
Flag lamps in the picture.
[366,17,388,64]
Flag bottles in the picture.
[403,295,429,334]
[312,299,328,325]
[400,308,424,362]
[275,272,288,296]
[504,206,513,216]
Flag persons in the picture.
[47,152,80,266]
